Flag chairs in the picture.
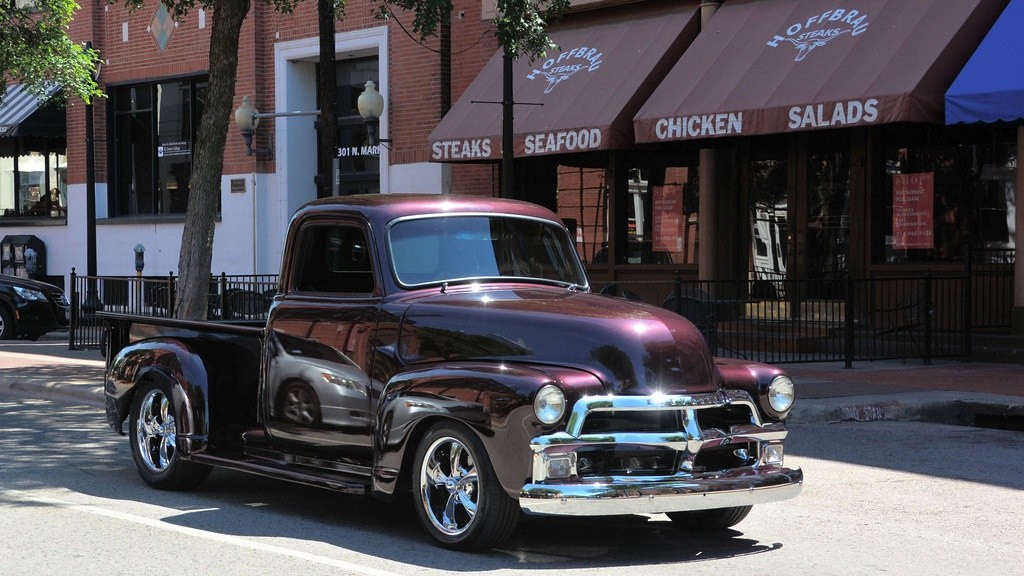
[150,285,277,320]
[598,286,717,357]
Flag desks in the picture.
[701,298,761,360]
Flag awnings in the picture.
[428,12,701,163]
[945,0,1024,125]
[0,81,65,137]
[632,0,1010,146]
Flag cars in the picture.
[0,271,70,343]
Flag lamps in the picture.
[357,74,393,151]
[235,95,273,162]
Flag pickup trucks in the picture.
[96,192,805,556]
